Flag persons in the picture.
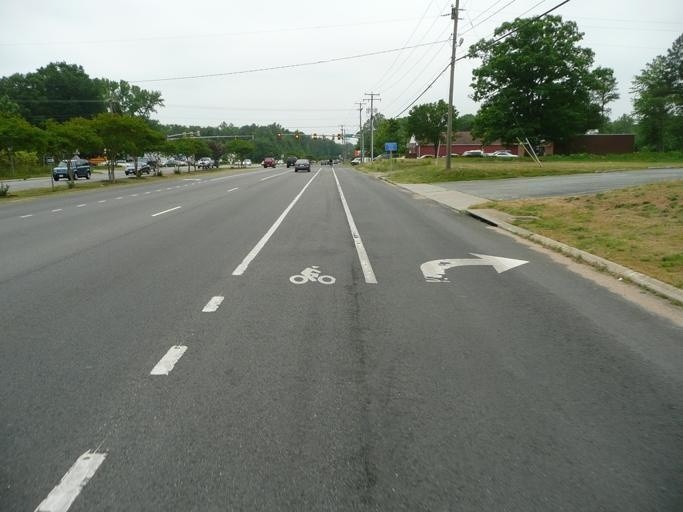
[328,158,333,167]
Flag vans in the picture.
[53,159,91,181]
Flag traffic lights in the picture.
[338,134,341,140]
[295,131,299,139]
[277,133,281,140]
[313,133,317,141]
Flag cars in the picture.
[261,157,340,172]
[242,158,252,166]
[125,162,151,175]
[351,150,519,166]
[198,157,219,168]
